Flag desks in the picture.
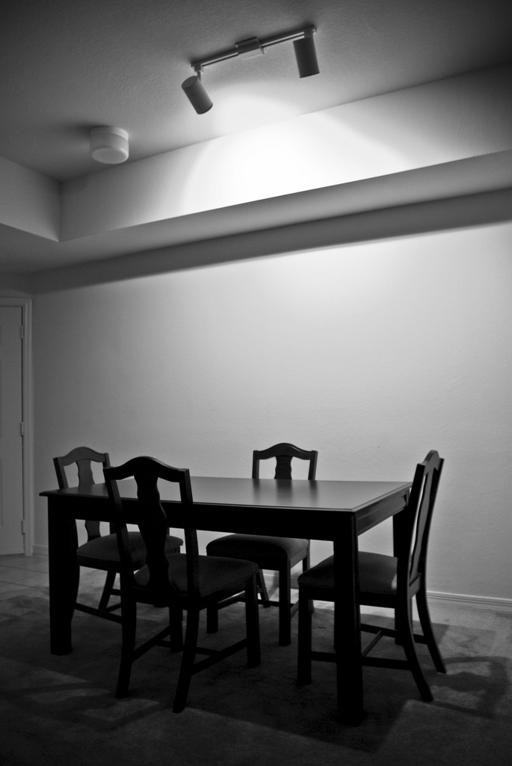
[38,476,413,726]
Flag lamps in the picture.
[181,24,321,115]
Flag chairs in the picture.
[206,443,318,647]
[52,446,184,625]
[103,456,261,715]
[297,450,448,704]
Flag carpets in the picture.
[0,569,512,766]
